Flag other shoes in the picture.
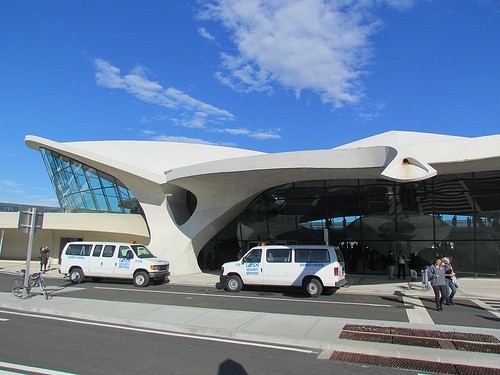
[437,307,442,311]
[450,299,455,305]
[446,302,452,305]
[440,306,444,310]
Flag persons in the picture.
[342,218,346,230]
[344,243,357,251]
[467,217,471,227]
[237,248,243,261]
[325,220,330,229]
[395,250,407,280]
[406,254,417,281]
[387,251,397,280]
[428,258,446,311]
[472,217,482,227]
[40,246,50,271]
[493,218,497,227]
[441,256,457,306]
[452,216,457,228]
[130,240,137,257]
[367,248,376,270]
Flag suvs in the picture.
[219,245,347,296]
[58,242,169,287]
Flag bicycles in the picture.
[11,268,48,299]
